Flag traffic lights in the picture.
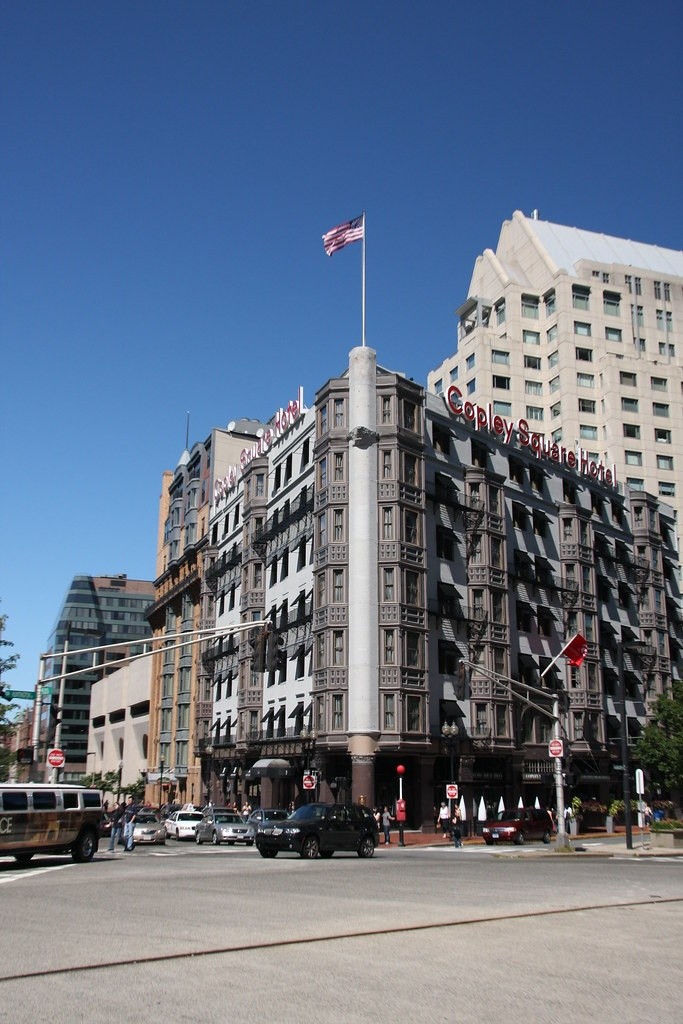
[563,738,574,757]
[267,632,285,672]
[565,774,577,786]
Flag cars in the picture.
[99,802,256,846]
[245,809,289,846]
[255,802,381,859]
[482,807,553,846]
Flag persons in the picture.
[644,803,653,828]
[546,807,558,832]
[288,804,292,814]
[122,797,136,851]
[107,803,123,851]
[242,802,252,821]
[226,802,239,810]
[437,802,451,839]
[565,805,573,833]
[451,804,462,848]
[381,807,395,845]
[373,807,381,827]
[102,800,109,810]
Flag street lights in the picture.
[158,752,166,809]
[116,760,124,803]
[299,723,319,804]
[194,744,215,806]
[441,720,460,843]
[616,641,647,850]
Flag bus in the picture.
[0,783,105,863]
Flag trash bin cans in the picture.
[654,810,664,822]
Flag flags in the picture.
[322,215,363,256]
[564,634,588,667]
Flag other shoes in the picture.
[442,836,446,838]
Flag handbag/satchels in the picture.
[437,823,441,828]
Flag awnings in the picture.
[260,702,311,722]
[263,588,313,619]
[212,630,232,644]
[215,538,244,564]
[250,759,296,778]
[289,644,313,660]
[214,582,241,603]
[210,671,238,686]
[265,533,314,569]
[265,483,314,524]
[209,717,237,732]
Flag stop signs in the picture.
[303,775,316,789]
[548,739,564,758]
[46,749,65,768]
[446,784,458,799]
